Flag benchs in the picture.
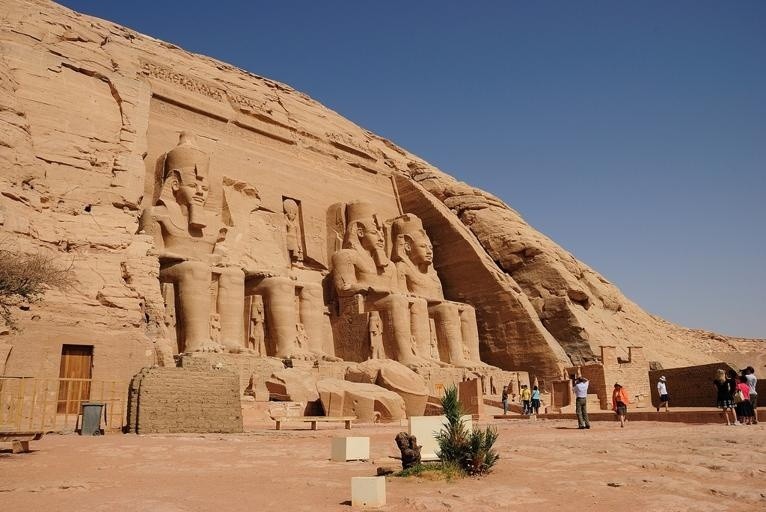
[271,415,357,431]
[0,430,44,454]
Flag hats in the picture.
[660,376,665,381]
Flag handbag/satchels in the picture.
[733,384,745,404]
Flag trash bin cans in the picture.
[81,403,107,436]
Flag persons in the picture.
[333,200,433,367]
[281,198,304,263]
[530,386,540,414]
[611,382,629,428]
[714,366,759,424]
[656,375,672,412]
[573,377,591,429]
[387,209,494,370]
[520,385,525,416]
[147,145,244,363]
[523,384,531,416]
[502,385,509,414]
